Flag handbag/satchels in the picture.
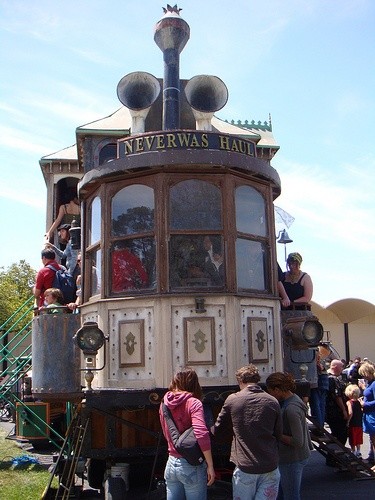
[174,426,206,466]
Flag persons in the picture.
[265,372,313,500]
[0,364,32,419]
[30,185,97,316]
[158,366,215,500]
[111,237,290,307]
[327,356,375,464]
[281,253,312,311]
[211,362,284,500]
[308,351,329,437]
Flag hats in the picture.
[59,224,70,230]
[289,252,302,265]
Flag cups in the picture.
[27,277,35,288]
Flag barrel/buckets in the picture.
[111,463,130,491]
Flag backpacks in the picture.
[44,265,75,304]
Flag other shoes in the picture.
[309,430,323,436]
[326,462,339,467]
[356,451,362,458]
[365,454,375,465]
